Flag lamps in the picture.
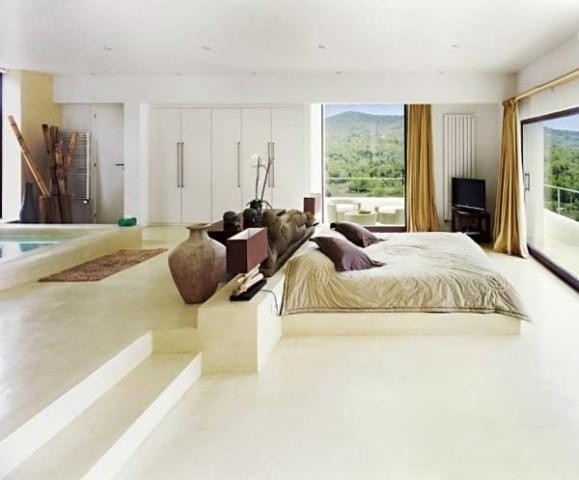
[302,192,322,227]
[225,225,270,302]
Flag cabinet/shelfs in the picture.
[451,208,492,244]
[149,108,211,224]
[240,108,309,213]
[211,108,241,224]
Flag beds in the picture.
[279,222,533,324]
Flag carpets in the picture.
[33,247,170,283]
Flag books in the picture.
[463,224,480,234]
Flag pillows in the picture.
[328,219,388,249]
[309,235,389,272]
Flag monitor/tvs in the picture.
[451,177,486,213]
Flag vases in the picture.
[223,199,263,224]
[169,222,229,307]
[19,181,38,224]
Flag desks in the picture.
[207,207,288,244]
